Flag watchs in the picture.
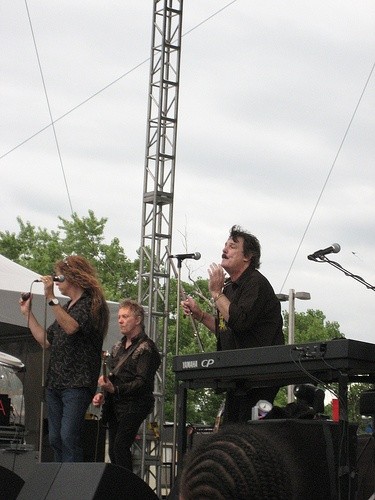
[48,299,60,305]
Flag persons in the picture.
[93,300,161,473]
[19,256,109,462]
[178,424,293,500]
[181,225,285,424]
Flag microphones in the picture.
[34,274,65,283]
[169,252,202,259]
[306,243,341,260]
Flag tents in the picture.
[0,254,155,480]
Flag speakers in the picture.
[16,460,159,499]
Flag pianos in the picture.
[171,338,375,500]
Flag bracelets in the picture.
[199,311,205,323]
[214,293,224,302]
